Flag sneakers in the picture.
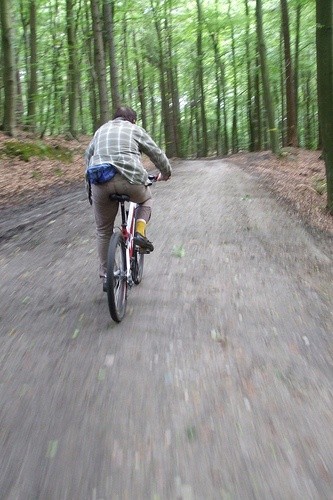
[134,236,154,252]
[102,277,116,292]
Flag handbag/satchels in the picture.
[87,163,115,185]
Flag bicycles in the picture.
[101,173,164,322]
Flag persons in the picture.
[83,104,173,293]
